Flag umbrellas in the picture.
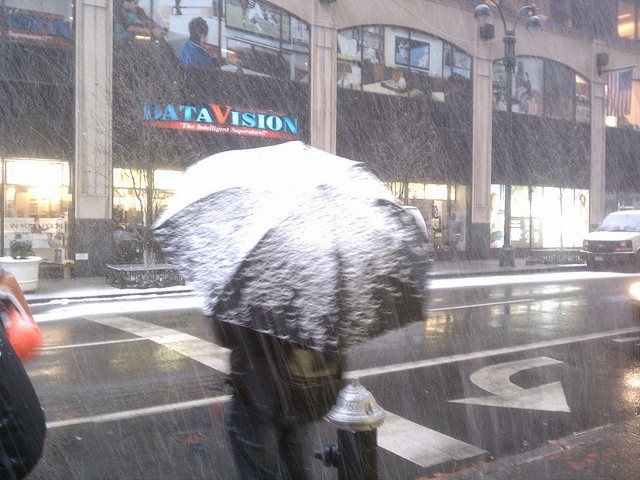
[148,139,430,355]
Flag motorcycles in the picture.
[430,225,445,260]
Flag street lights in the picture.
[475,0,542,268]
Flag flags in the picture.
[606,69,633,119]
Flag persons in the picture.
[213,316,342,480]
[29,217,48,234]
[516,73,533,113]
[0,319,47,479]
[446,212,459,262]
[177,15,221,100]
[114,0,172,64]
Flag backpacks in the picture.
[226,337,343,424]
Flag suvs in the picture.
[581,210,639,269]
